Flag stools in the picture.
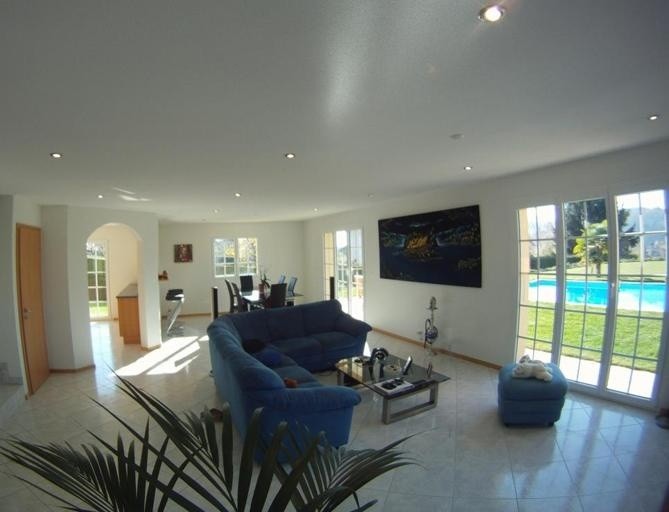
[497,363,568,427]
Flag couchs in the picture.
[207,299,373,466]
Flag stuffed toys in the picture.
[512,355,554,381]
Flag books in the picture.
[374,377,414,397]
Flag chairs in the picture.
[224,274,298,312]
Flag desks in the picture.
[116,282,141,346]
